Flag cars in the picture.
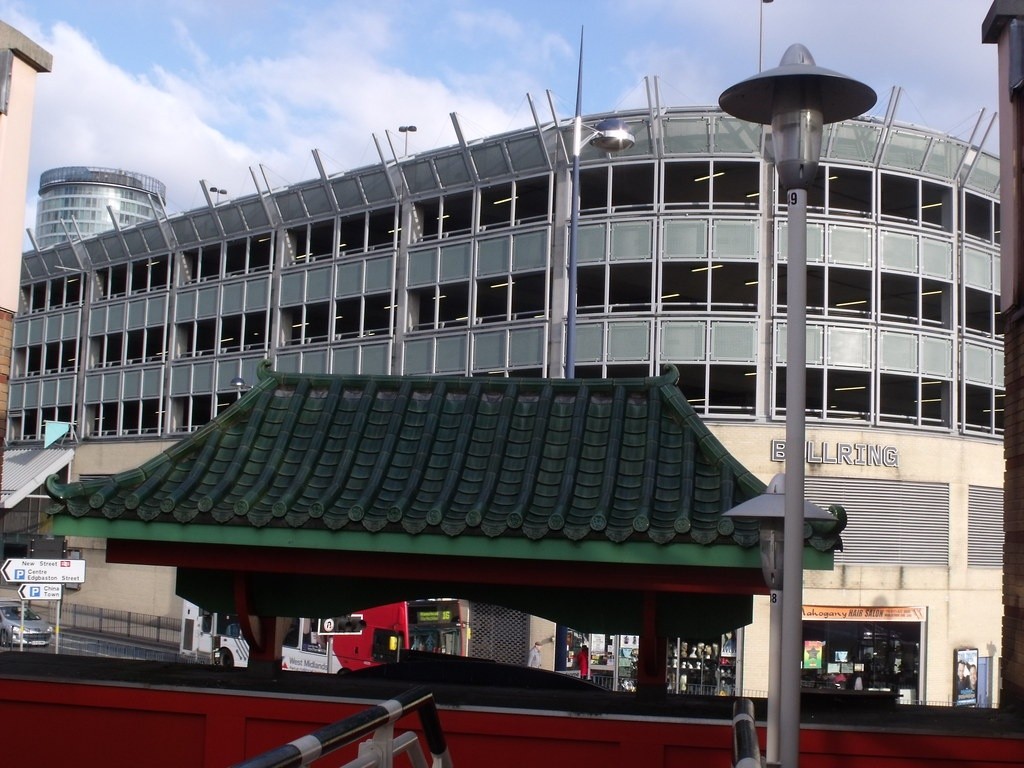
[0,599,54,647]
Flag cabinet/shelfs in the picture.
[668,652,718,685]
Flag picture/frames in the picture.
[953,647,978,708]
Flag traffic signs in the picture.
[0,559,86,583]
[17,583,61,600]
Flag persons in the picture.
[578,644,593,681]
[956,661,976,699]
[527,642,543,668]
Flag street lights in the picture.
[723,470,839,768]
[719,41,878,768]
[566,118,638,380]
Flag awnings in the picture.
[0,448,73,508]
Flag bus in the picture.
[179,597,467,676]
